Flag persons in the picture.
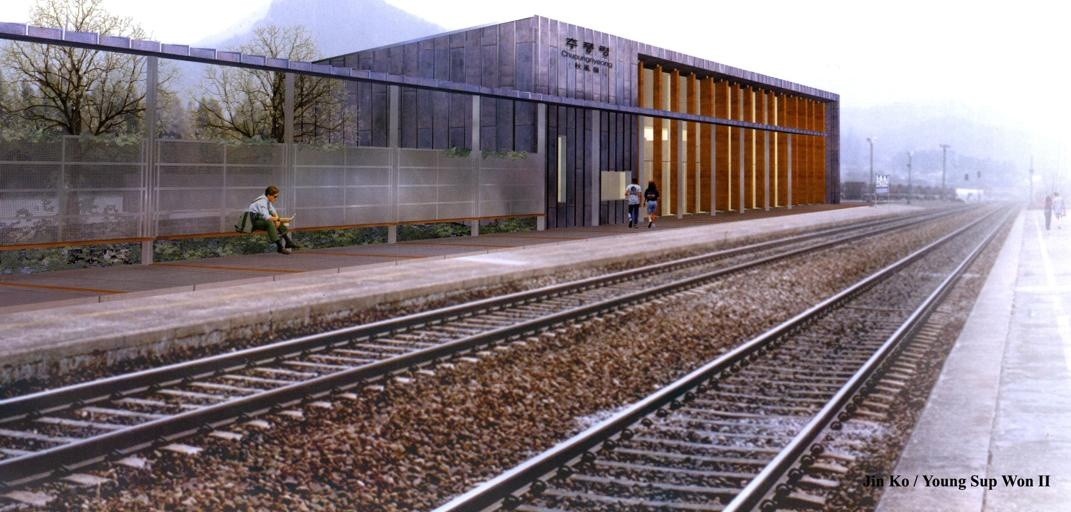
[248,185,302,254]
[1052,192,1067,229]
[1043,195,1053,230]
[643,181,662,229]
[625,177,643,229]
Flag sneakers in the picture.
[285,241,304,247]
[628,218,656,228]
[277,247,292,254]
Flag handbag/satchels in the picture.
[235,212,257,233]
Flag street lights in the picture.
[865,136,947,199]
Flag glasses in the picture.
[274,195,277,198]
